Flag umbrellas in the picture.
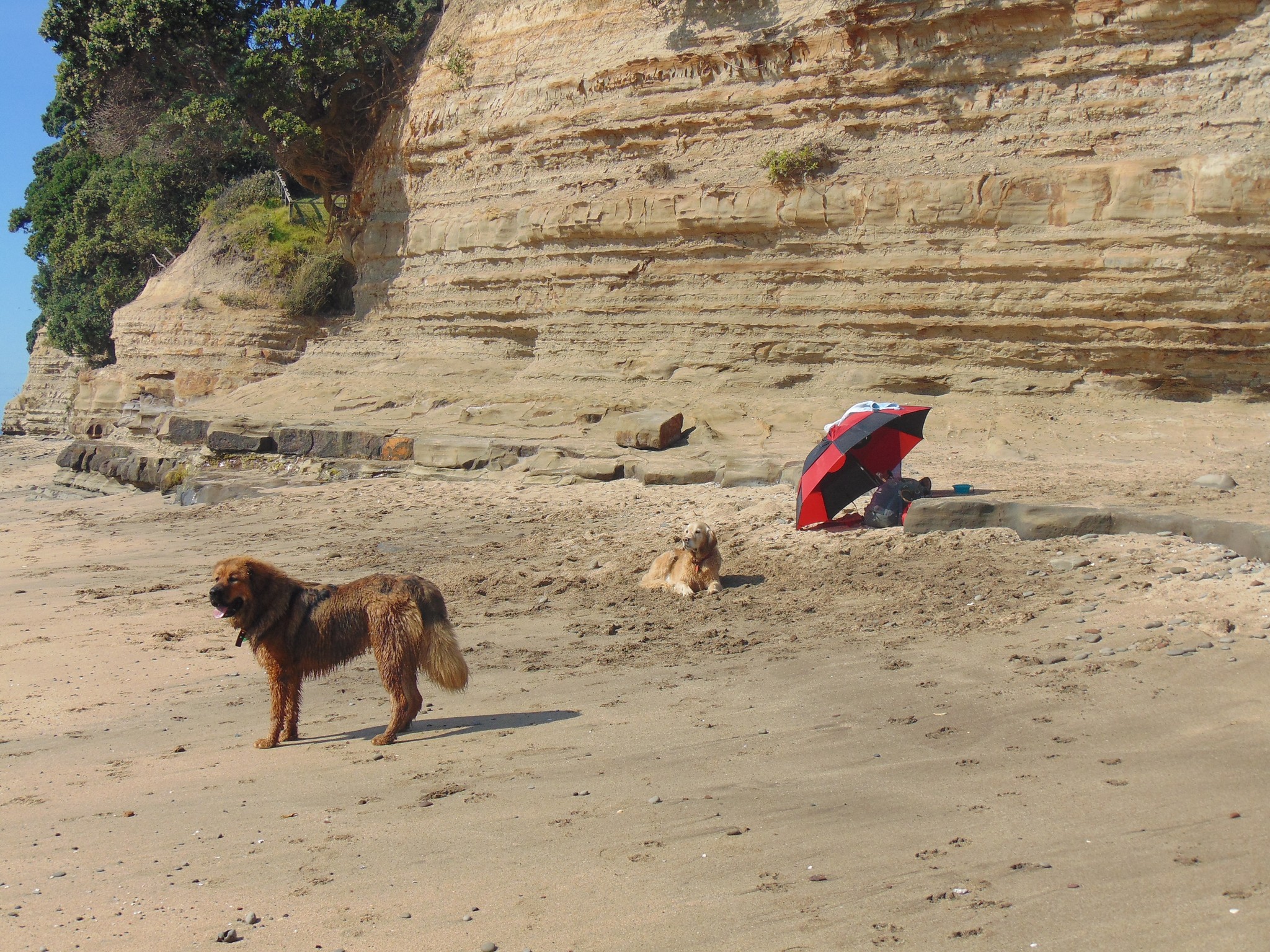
[795,406,933,530]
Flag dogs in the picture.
[635,522,724,596]
[208,553,473,750]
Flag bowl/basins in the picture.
[952,484,971,494]
